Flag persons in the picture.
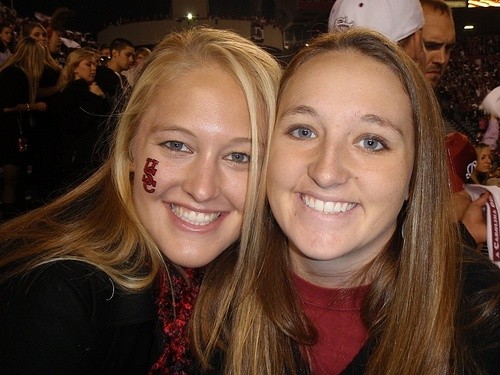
[0,24,285,375]
[0,6,152,224]
[329,0,500,267]
[188,28,500,375]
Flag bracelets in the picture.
[27,103,32,112]
